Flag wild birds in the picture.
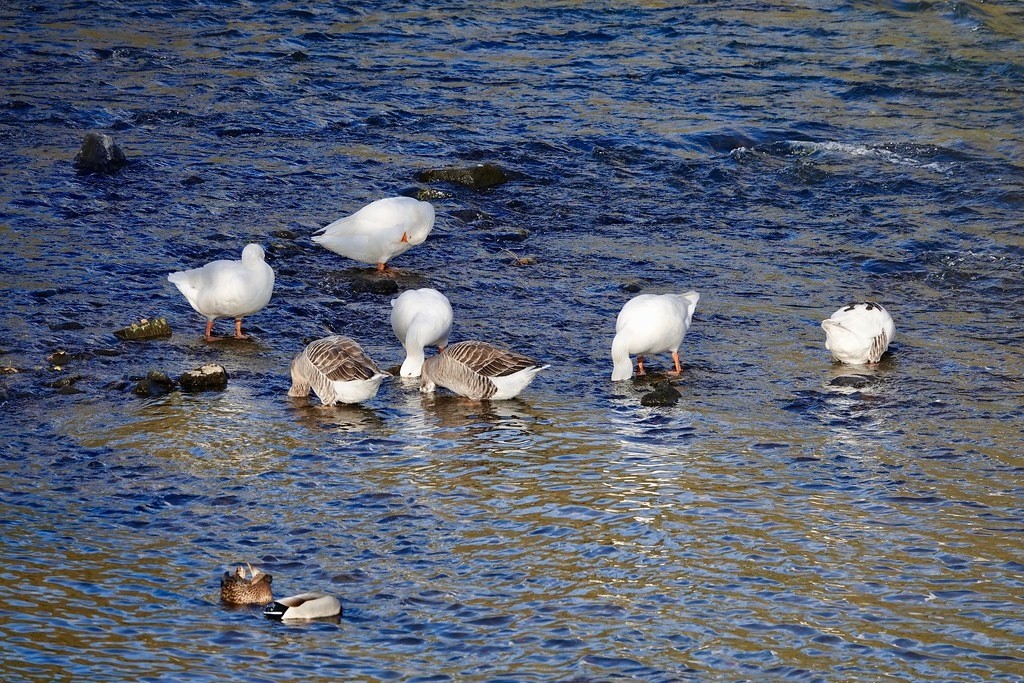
[287,287,553,413]
[310,195,436,271]
[819,296,897,366]
[219,560,343,620]
[610,288,701,383]
[167,241,277,342]
